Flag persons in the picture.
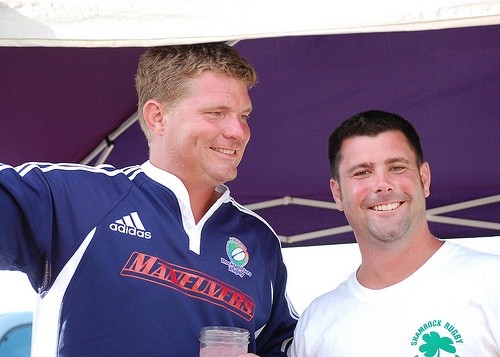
[285,109,500,357]
[0,43,303,357]
